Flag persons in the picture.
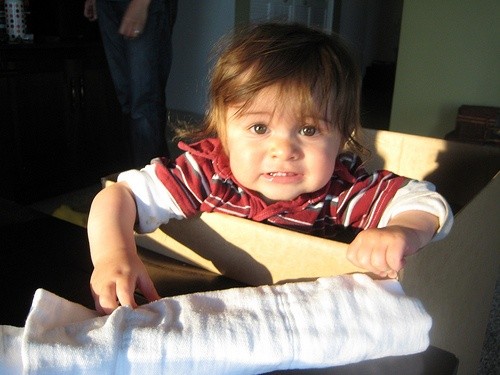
[84,0,179,170]
[86,20,454,317]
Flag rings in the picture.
[134,30,140,33]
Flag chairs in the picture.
[98,122,500,375]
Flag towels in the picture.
[0,273,434,375]
